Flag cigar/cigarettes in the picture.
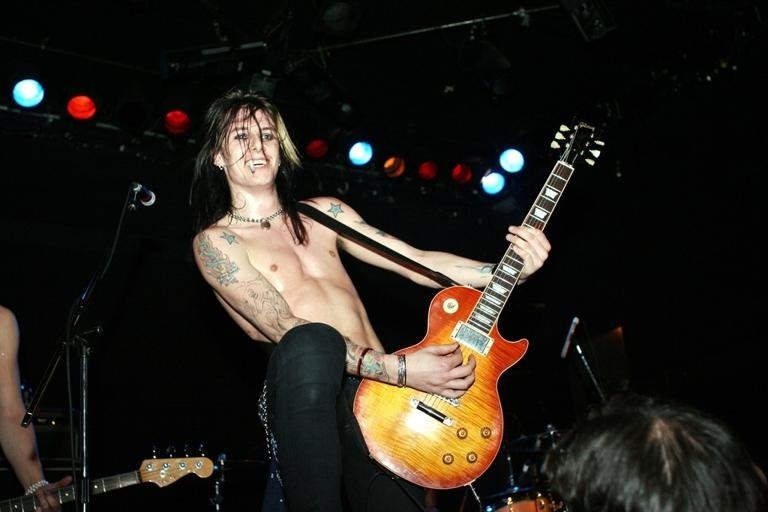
[249,163,256,173]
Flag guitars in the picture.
[0,445,214,510]
[353,120,605,490]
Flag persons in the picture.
[190,87,551,512]
[1,306,74,512]
[539,391,768,512]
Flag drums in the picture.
[482,488,569,512]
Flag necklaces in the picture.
[226,208,284,232]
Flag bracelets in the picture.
[25,479,48,496]
[356,347,374,381]
[396,355,407,386]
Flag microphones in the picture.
[133,181,157,206]
[559,316,582,360]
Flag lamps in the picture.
[12,76,525,197]
